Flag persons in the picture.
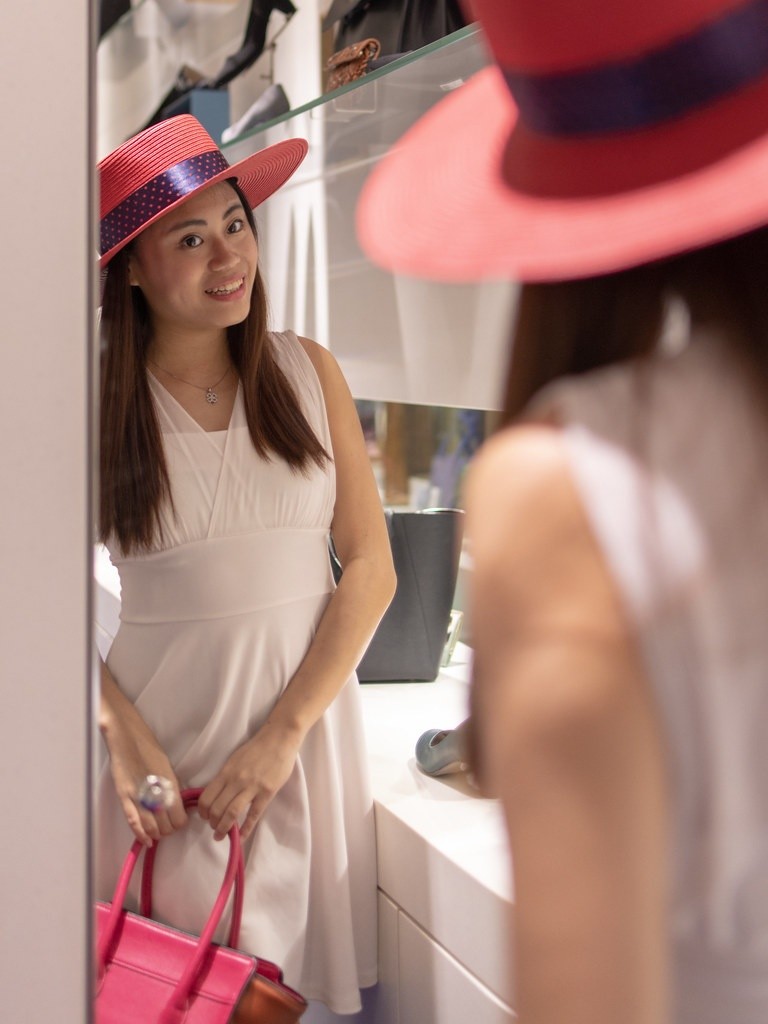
[356,0,768,1024]
[97,113,398,1024]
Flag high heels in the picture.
[415,716,475,791]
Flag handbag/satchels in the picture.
[94,788,309,1024]
[325,38,381,91]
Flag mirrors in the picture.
[87,0,517,1024]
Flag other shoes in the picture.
[219,84,290,144]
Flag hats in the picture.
[357,0,768,283]
[97,112,307,313]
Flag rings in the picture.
[139,775,173,813]
